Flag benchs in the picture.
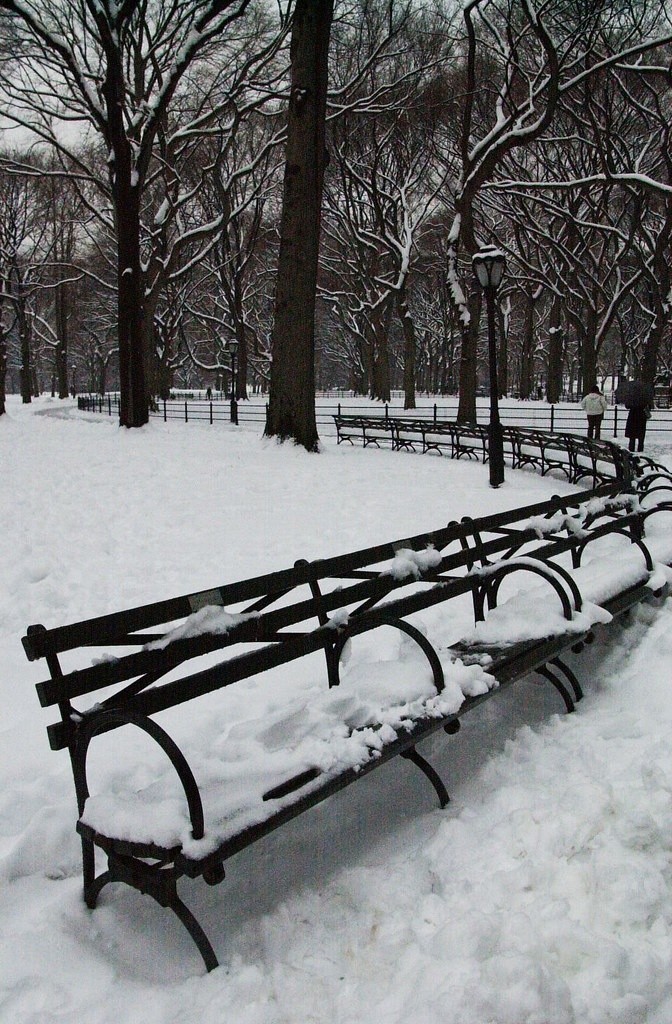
[20,416,672,971]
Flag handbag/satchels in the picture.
[643,408,652,419]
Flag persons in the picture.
[581,385,607,439]
[481,388,485,397]
[625,380,654,452]
[72,388,76,399]
[538,386,544,400]
[207,387,211,399]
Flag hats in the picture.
[591,386,599,392]
